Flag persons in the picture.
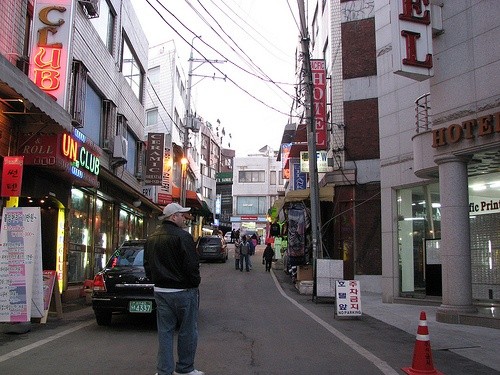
[230,228,261,272]
[143,203,205,375]
[263,243,275,272]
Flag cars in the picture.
[224,232,234,243]
[196,235,229,263]
[91,239,200,330]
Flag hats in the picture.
[162,203,191,219]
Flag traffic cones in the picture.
[399,312,442,375]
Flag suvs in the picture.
[242,229,262,245]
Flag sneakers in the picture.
[173,369,205,375]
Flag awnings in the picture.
[276,186,335,218]
